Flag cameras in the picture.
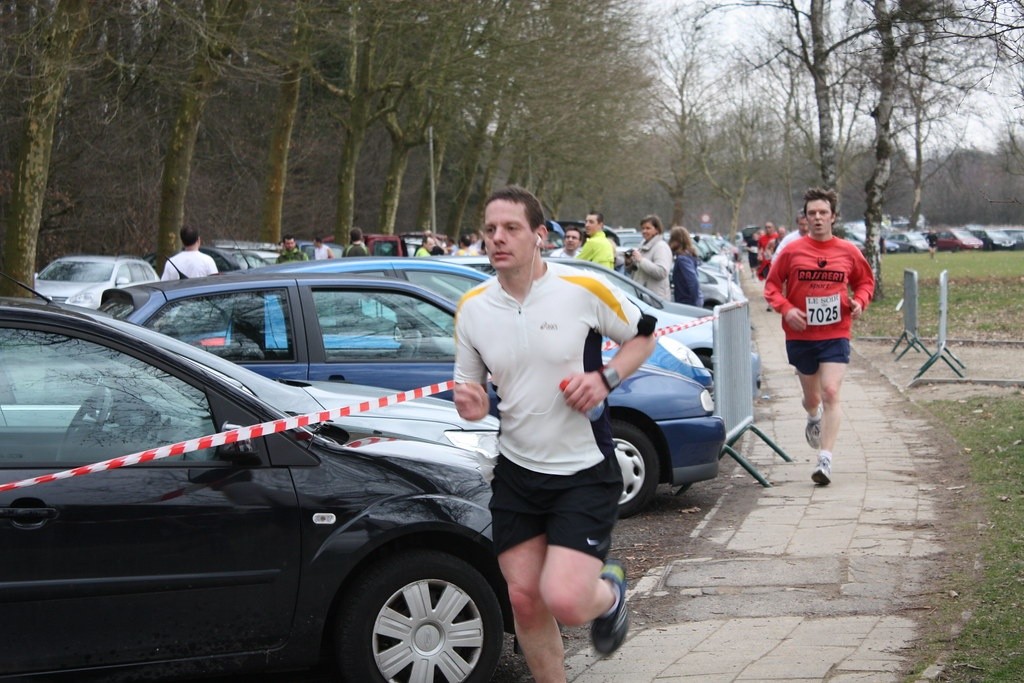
[625,249,634,258]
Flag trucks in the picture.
[321,232,449,257]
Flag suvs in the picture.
[34,255,161,312]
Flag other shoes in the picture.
[767,305,773,312]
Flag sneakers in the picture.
[592,559,630,656]
[811,458,831,486]
[805,405,823,448]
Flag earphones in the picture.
[537,234,541,246]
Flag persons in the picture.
[342,228,368,257]
[160,225,218,281]
[414,229,486,258]
[313,238,334,260]
[550,211,625,274]
[623,215,672,300]
[275,235,308,264]
[452,186,656,683]
[764,187,875,484]
[746,215,810,311]
[667,227,703,308]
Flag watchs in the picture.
[598,367,622,392]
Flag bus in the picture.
[142,249,270,280]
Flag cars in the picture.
[0,294,521,683]
[187,255,712,384]
[179,242,345,276]
[95,275,727,519]
[0,353,502,458]
[884,242,899,254]
[934,230,983,252]
[1005,230,1024,250]
[972,230,1016,250]
[546,221,747,310]
[440,257,761,396]
[887,232,931,254]
